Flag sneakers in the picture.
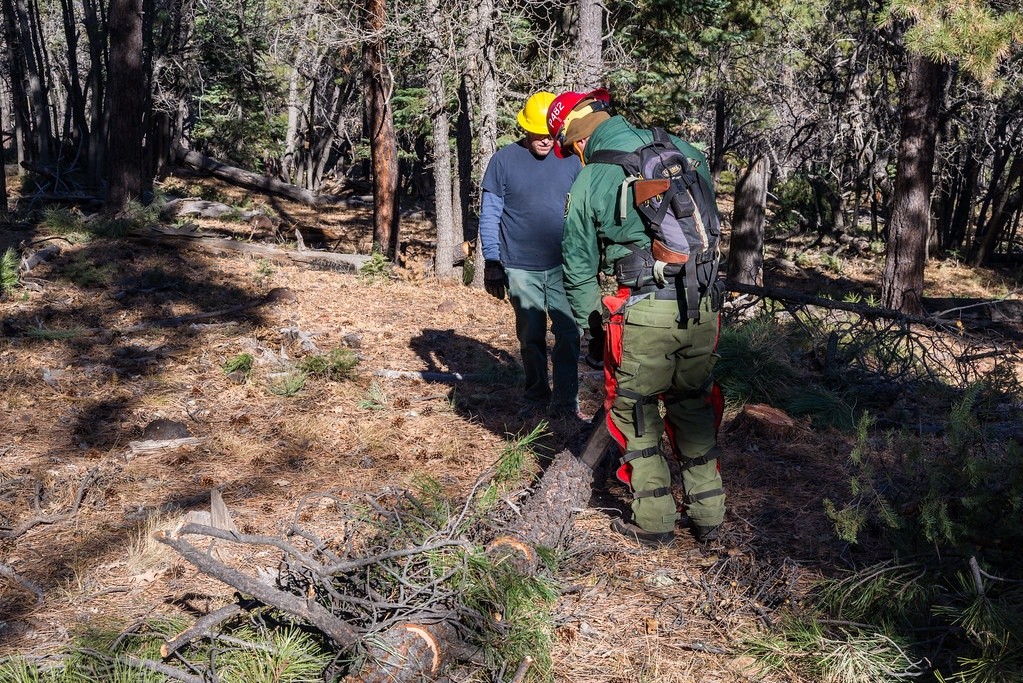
[612,517,673,548]
[548,397,595,424]
[512,404,543,425]
[698,526,719,539]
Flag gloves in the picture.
[484,260,510,300]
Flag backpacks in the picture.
[590,125,725,318]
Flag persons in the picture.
[481,92,584,413]
[547,88,725,542]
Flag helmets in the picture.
[547,88,609,159]
[518,90,557,134]
